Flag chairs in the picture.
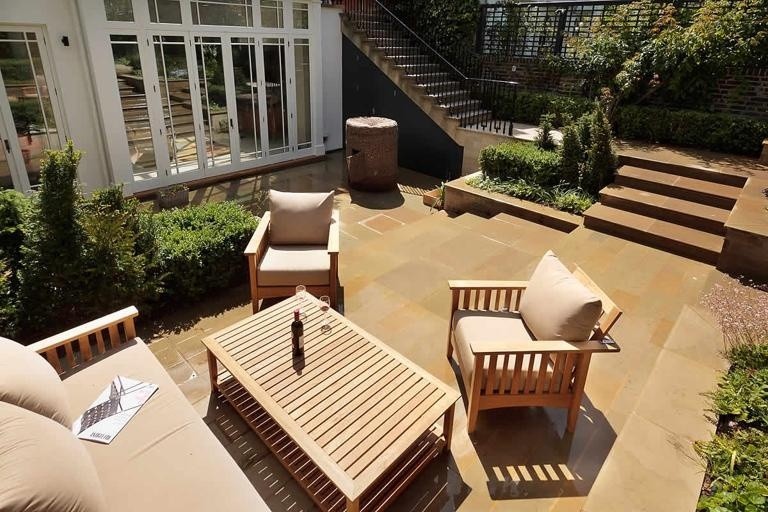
[445,262,621,434]
[243,211,340,314]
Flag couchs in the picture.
[25,305,271,512]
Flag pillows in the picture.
[266,188,336,245]
[0,400,109,512]
[518,249,602,377]
[0,336,74,430]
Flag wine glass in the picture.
[295,285,307,315]
[318,295,331,325]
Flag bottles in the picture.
[291,308,304,353]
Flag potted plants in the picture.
[156,183,190,210]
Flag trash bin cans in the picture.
[345,116,399,193]
